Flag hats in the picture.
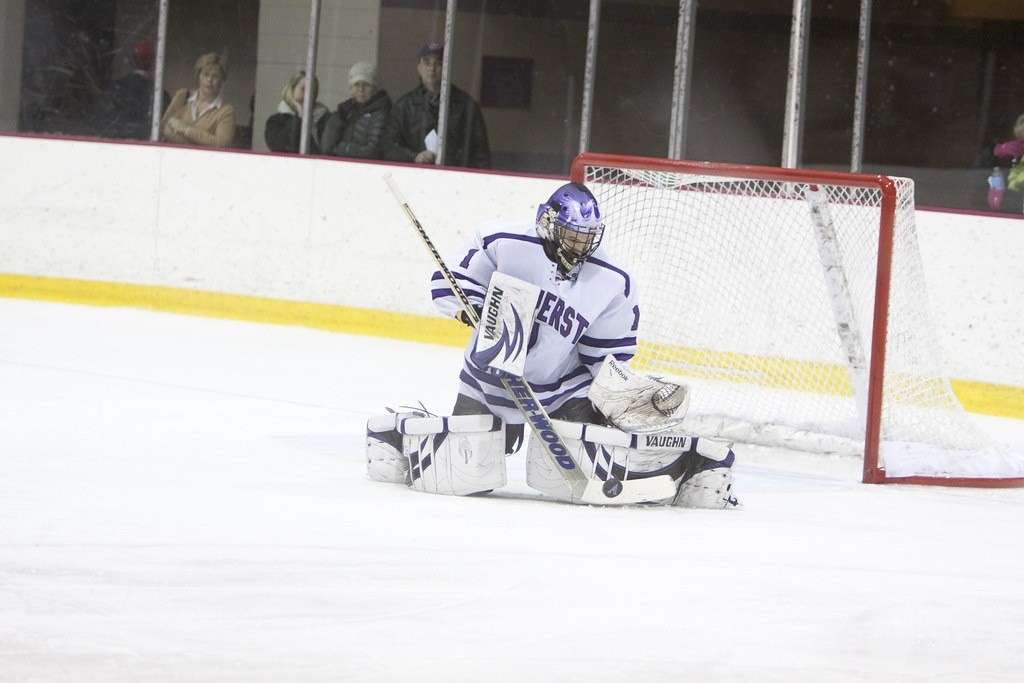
[418,41,443,57]
[346,61,388,91]
[132,43,154,63]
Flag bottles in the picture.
[988,166,1004,212]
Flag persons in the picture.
[161,53,240,148]
[971,112,1024,212]
[321,59,393,160]
[381,44,493,169]
[366,183,743,512]
[106,41,171,138]
[266,71,333,155]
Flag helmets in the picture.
[536,182,606,274]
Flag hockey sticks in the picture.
[379,168,679,507]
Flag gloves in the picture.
[415,150,434,164]
